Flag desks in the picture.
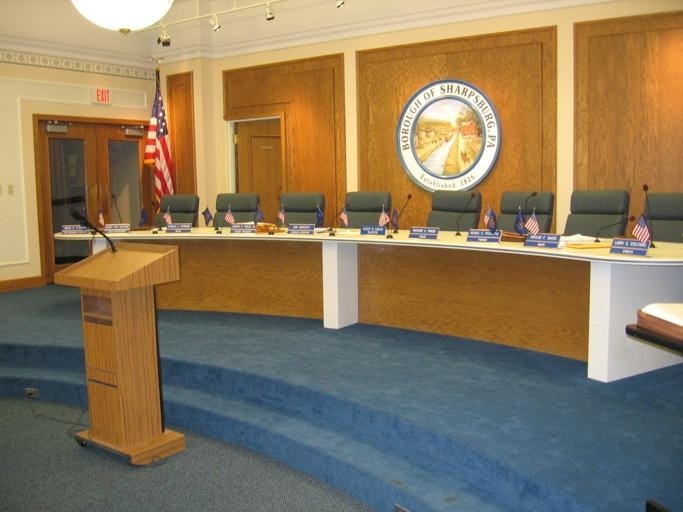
[52,224,683,383]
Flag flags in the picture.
[202,208,212,226]
[377,207,390,227]
[338,208,349,227]
[631,215,650,244]
[163,208,172,224]
[390,210,398,233]
[484,207,539,237]
[256,206,265,222]
[316,207,324,225]
[139,209,147,227]
[224,209,235,225]
[143,67,174,216]
[80,211,87,226]
[276,206,285,224]
[99,209,105,226]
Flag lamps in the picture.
[157,0,350,48]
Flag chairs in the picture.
[212,193,260,228]
[563,191,631,239]
[642,191,683,244]
[344,191,392,229]
[156,195,200,227]
[496,190,554,235]
[279,192,326,228]
[426,189,482,233]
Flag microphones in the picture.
[109,191,126,224]
[67,205,118,253]
[328,202,352,237]
[592,212,637,245]
[641,181,658,250]
[524,188,538,217]
[454,190,476,237]
[387,194,413,241]
